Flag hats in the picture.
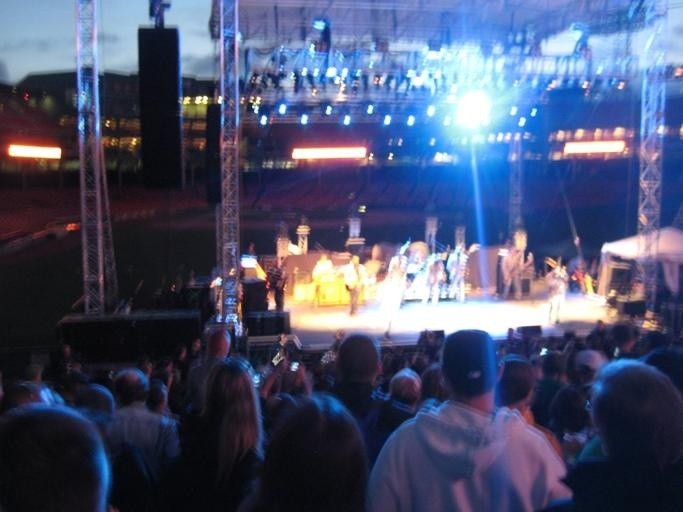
[444,330,496,393]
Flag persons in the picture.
[385,248,468,308]
[311,253,333,282]
[267,257,289,312]
[344,256,367,315]
[2,329,680,508]
[546,265,569,324]
[501,248,523,300]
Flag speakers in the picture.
[247,312,291,336]
[516,326,541,337]
[615,301,645,317]
[242,279,268,324]
